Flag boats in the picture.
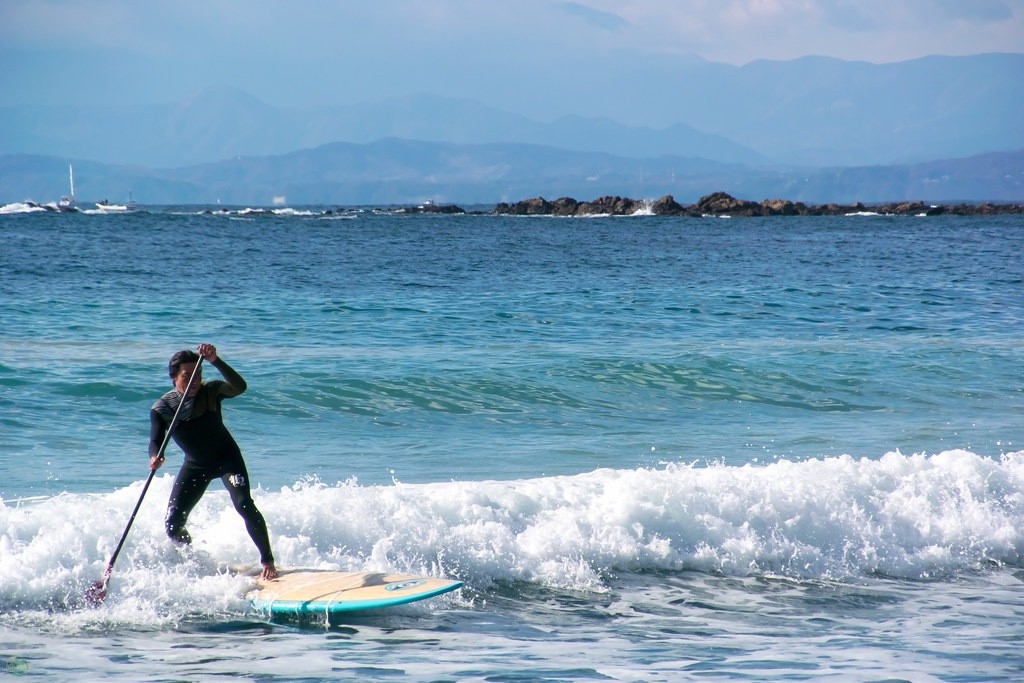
[24,200,58,211]
[76,199,142,215]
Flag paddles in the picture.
[86,355,204,607]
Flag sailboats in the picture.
[56,164,78,213]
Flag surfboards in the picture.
[213,566,465,615]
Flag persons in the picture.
[148,344,277,580]
